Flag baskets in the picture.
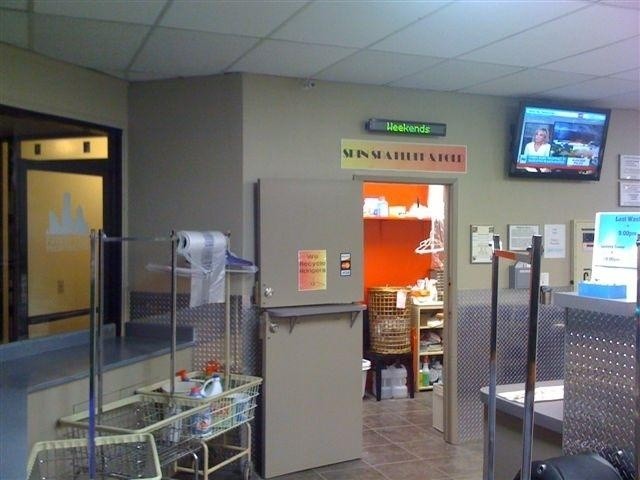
[25,370,263,480]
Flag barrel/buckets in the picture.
[392,359,408,398]
[200,374,223,396]
[372,364,391,399]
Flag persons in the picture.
[524,128,551,156]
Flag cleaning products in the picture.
[189,389,211,435]
[174,370,192,384]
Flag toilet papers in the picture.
[176,230,228,308]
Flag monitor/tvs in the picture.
[508,100,611,181]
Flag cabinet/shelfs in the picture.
[408,302,447,393]
